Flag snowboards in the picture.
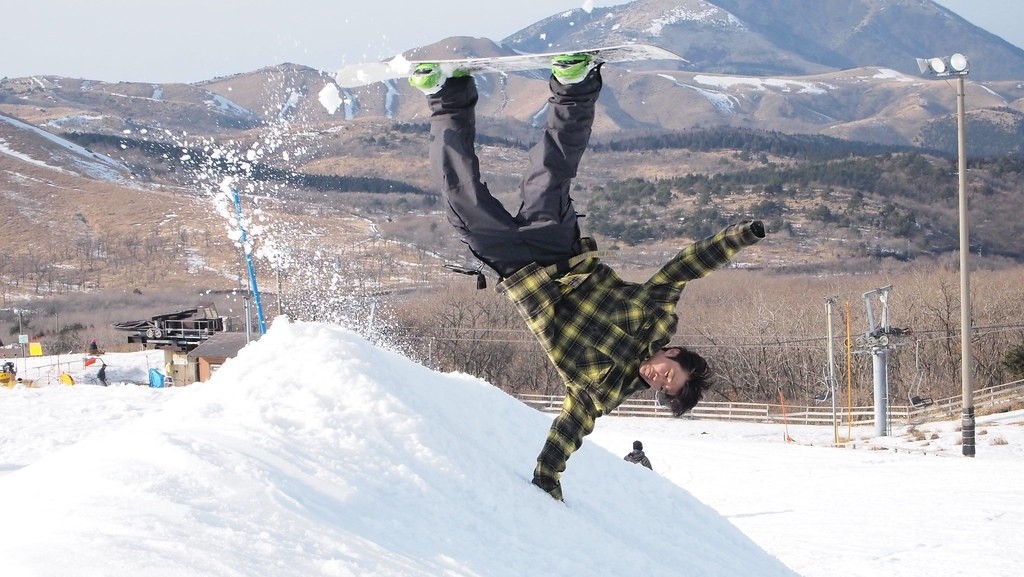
[335,41,693,90]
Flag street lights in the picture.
[916,52,976,457]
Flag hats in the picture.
[633,441,643,451]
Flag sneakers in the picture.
[407,64,470,95]
[551,54,596,85]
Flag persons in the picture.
[409,52,765,500]
[97,364,107,386]
[165,360,175,387]
[623,440,653,471]
[89,338,98,354]
